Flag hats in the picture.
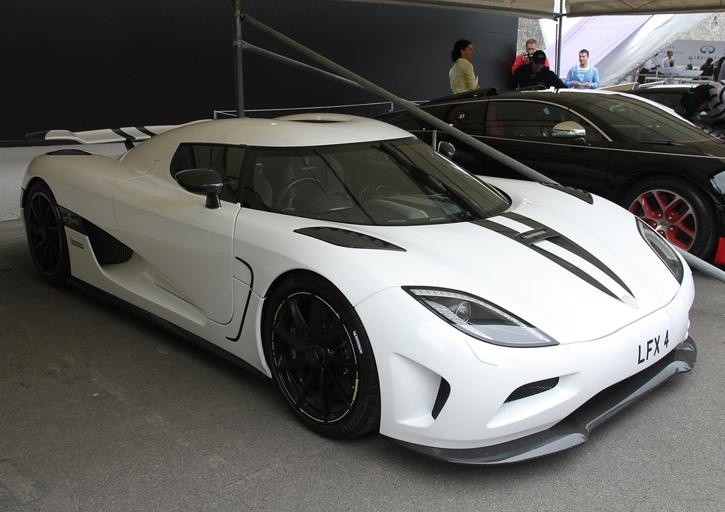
[533,50,546,63]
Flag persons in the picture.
[511,39,549,76]
[449,39,479,96]
[565,49,600,89]
[638,52,658,83]
[662,50,674,68]
[700,57,725,82]
[679,80,725,127]
[512,50,567,89]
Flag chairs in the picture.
[232,140,377,222]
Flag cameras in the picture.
[527,51,534,61]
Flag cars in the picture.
[605,75,723,135]
[18,109,699,467]
[374,88,725,271]
